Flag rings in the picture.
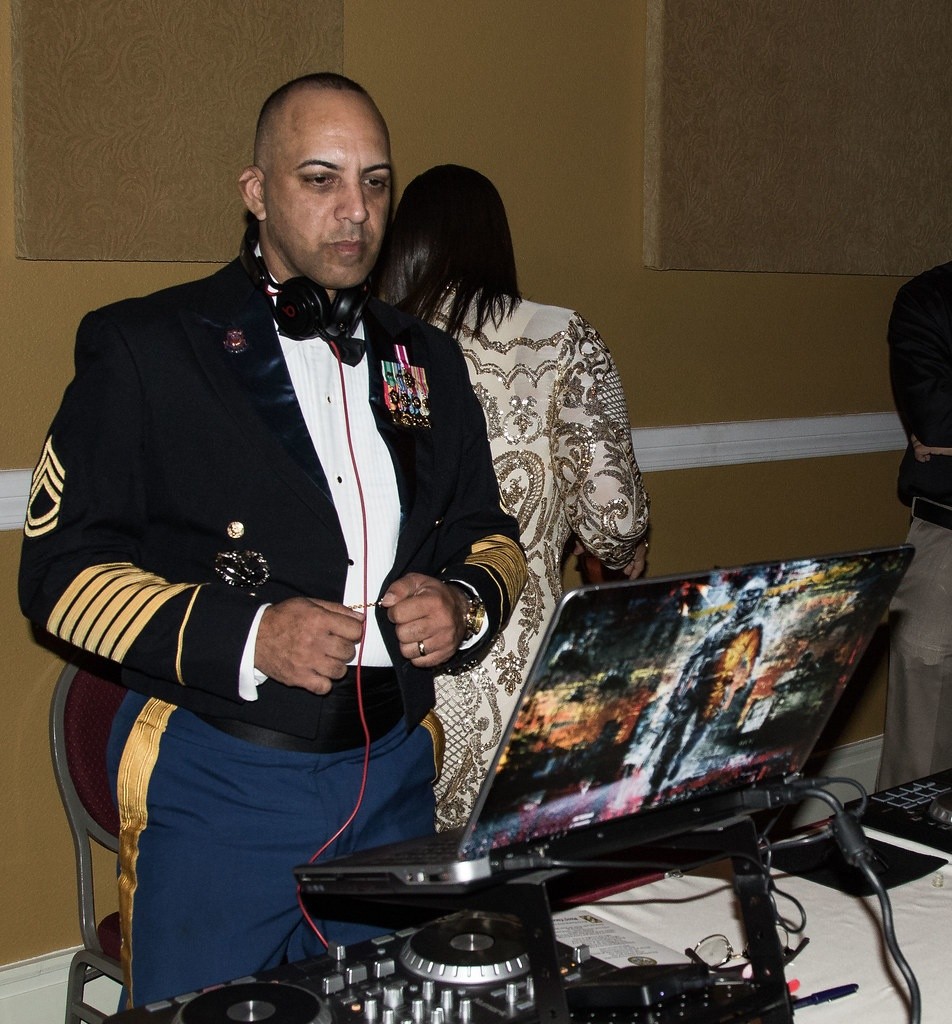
[418,641,426,656]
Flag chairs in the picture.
[49,661,129,1024]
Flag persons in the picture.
[363,164,647,834]
[18,73,527,1015]
[873,260,952,793]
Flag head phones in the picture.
[239,227,373,340]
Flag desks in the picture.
[98,768,952,1024]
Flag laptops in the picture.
[290,540,919,885]
